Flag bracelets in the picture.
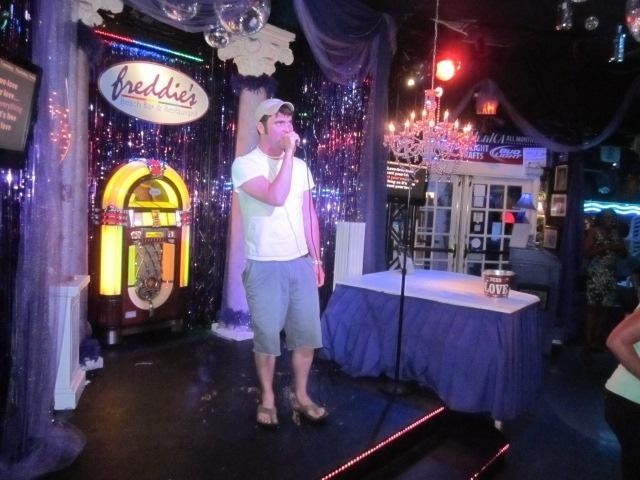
[313,259,323,266]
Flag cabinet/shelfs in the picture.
[48,274,91,411]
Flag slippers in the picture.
[255,401,281,428]
[292,401,330,421]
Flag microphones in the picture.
[295,140,303,148]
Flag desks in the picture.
[319,268,543,431]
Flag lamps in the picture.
[555,1,573,32]
[582,14,602,34]
[536,200,544,210]
[511,192,536,222]
[608,24,627,64]
[383,0,481,183]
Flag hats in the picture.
[253,98,294,124]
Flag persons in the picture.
[583,209,628,354]
[602,304,640,480]
[230,99,329,428]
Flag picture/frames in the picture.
[554,164,569,191]
[543,228,557,249]
[550,194,567,217]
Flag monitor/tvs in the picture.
[1,49,43,169]
